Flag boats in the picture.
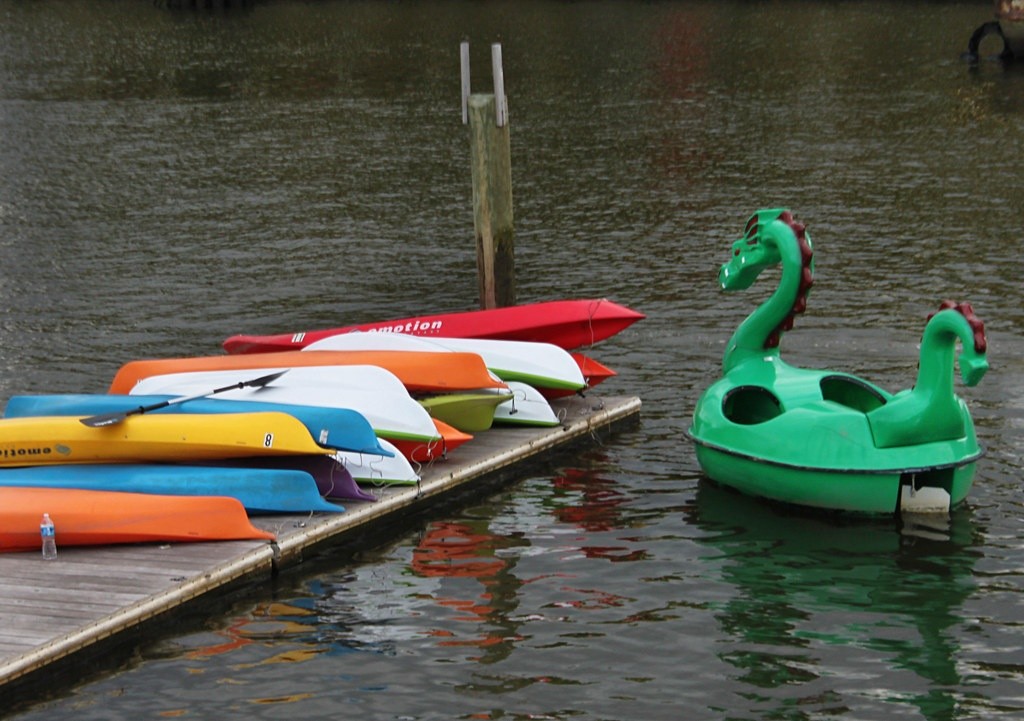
[682,209,992,512]
[1,411,325,454]
[0,484,279,552]
[683,475,983,719]
[4,331,617,503]
[223,293,646,354]
[1,466,346,512]
